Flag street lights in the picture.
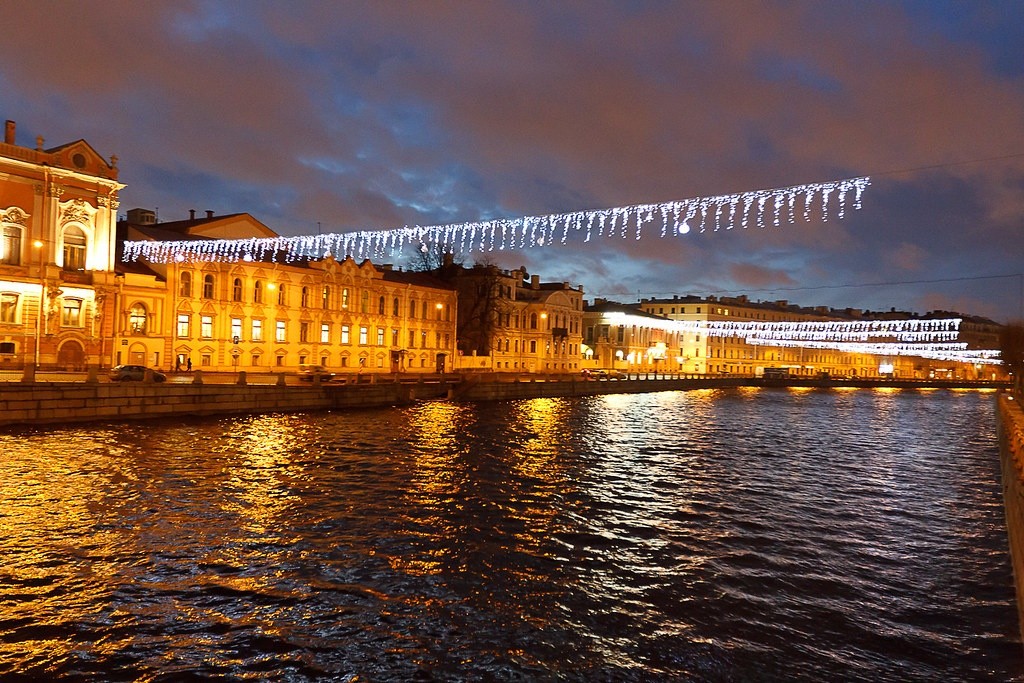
[32,240,44,382]
[267,283,276,370]
[541,313,547,375]
[436,303,443,353]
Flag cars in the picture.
[789,374,799,379]
[717,370,733,376]
[108,365,167,383]
[590,370,607,379]
[817,371,832,380]
[296,364,336,382]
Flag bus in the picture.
[755,366,789,379]
